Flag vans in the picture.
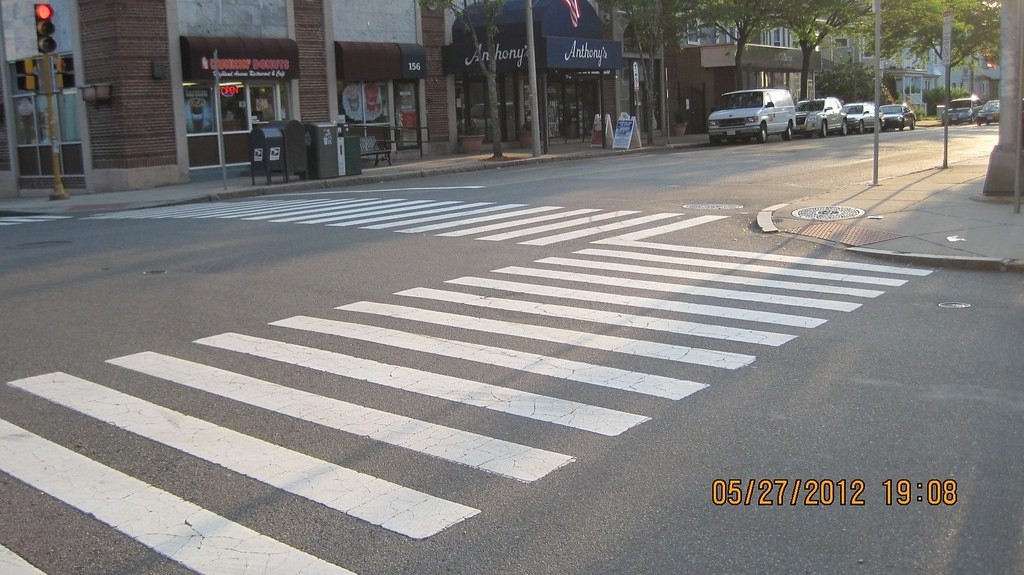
[708,89,796,145]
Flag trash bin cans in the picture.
[250,128,287,185]
[267,119,309,183]
[300,122,339,179]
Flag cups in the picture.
[365,86,378,111]
[189,98,205,130]
[348,94,360,111]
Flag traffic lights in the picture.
[34,3,57,52]
[15,59,41,91]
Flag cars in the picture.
[794,97,848,138]
[879,104,916,132]
[844,103,885,133]
[942,97,982,126]
[977,100,1000,126]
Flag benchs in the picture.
[360,136,392,167]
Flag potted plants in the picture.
[671,111,688,136]
[517,123,533,148]
[458,122,485,155]
